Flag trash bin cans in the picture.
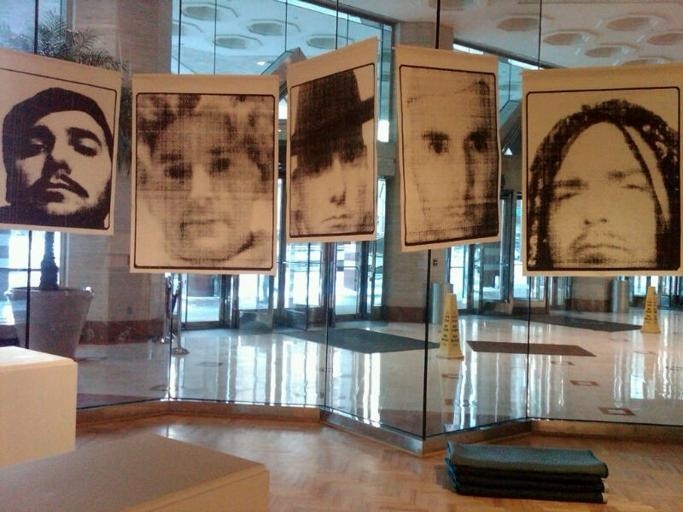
[432,282,453,324]
[611,280,629,312]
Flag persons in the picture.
[0,87,115,230]
[136,91,274,271]
[289,68,374,237]
[525,97,681,270]
[398,66,499,247]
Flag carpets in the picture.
[281,327,440,354]
[501,311,643,334]
[465,339,597,357]
[441,436,608,505]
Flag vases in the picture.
[4,285,97,359]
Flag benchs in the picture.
[0,430,272,511]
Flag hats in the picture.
[291,70,374,155]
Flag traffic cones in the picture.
[434,292,467,360]
[643,335,663,382]
[639,285,662,336]
[434,360,462,426]
